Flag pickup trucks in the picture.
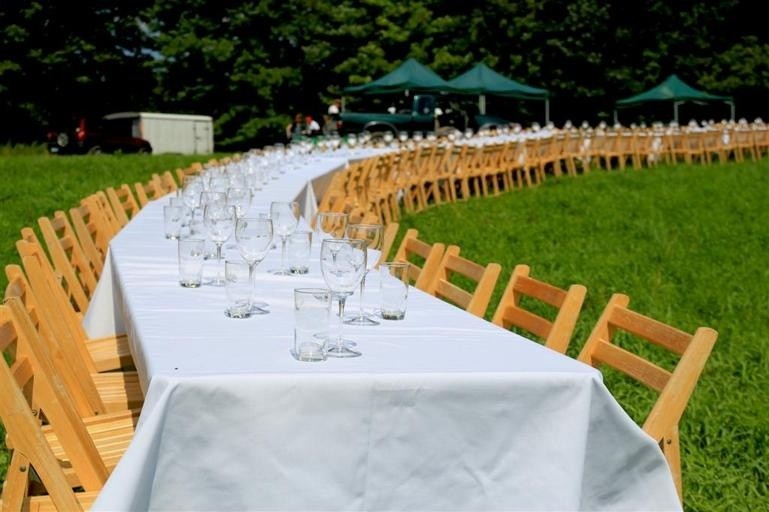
[331,89,516,140]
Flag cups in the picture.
[224,259,253,318]
[178,237,205,287]
[378,261,409,321]
[293,286,333,363]
[319,239,366,359]
[288,230,311,274]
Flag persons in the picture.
[285,113,312,145]
[322,114,343,137]
[327,99,341,116]
[304,115,320,137]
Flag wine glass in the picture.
[268,201,300,275]
[235,218,274,307]
[315,211,347,271]
[345,223,380,327]
[165,129,522,251]
[202,204,237,288]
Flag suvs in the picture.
[44,113,154,158]
